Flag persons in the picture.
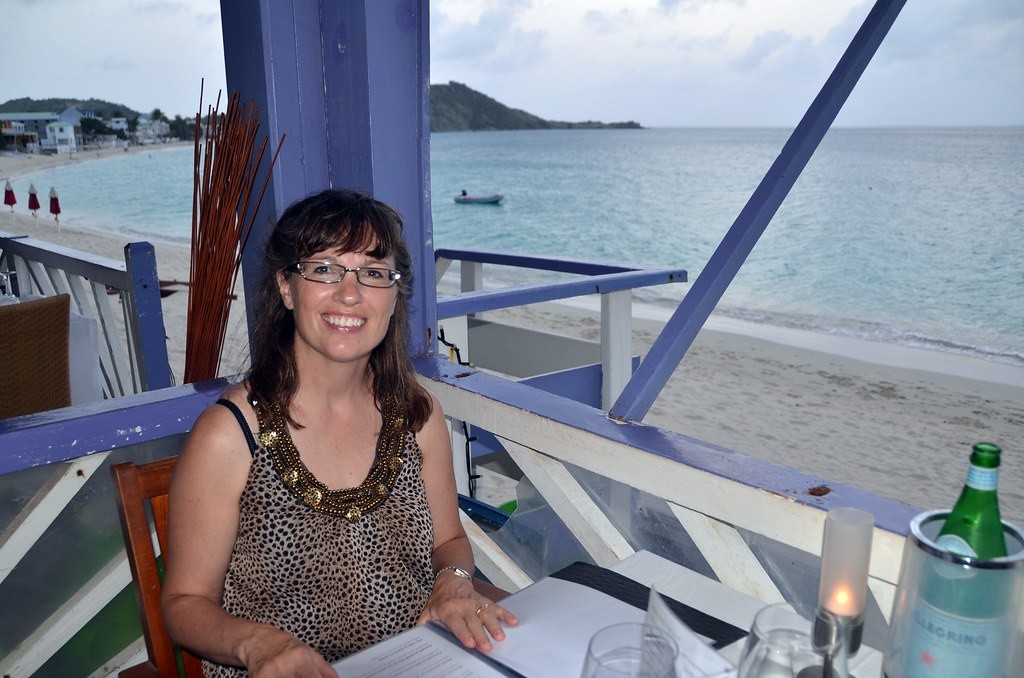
[161,191,518,678]
[462,189,467,196]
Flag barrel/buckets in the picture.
[879,509,1024,678]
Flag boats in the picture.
[454,193,503,203]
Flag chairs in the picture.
[110,453,205,678]
[0,293,72,420]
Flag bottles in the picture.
[904,442,1011,678]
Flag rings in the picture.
[476,603,489,614]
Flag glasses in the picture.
[285,262,402,289]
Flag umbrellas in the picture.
[48,186,61,232]
[4,179,17,221]
[28,182,40,227]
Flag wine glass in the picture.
[0,271,20,306]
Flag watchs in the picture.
[434,566,474,581]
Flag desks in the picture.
[611,549,883,678]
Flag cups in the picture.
[581,621,680,678]
[737,601,849,678]
